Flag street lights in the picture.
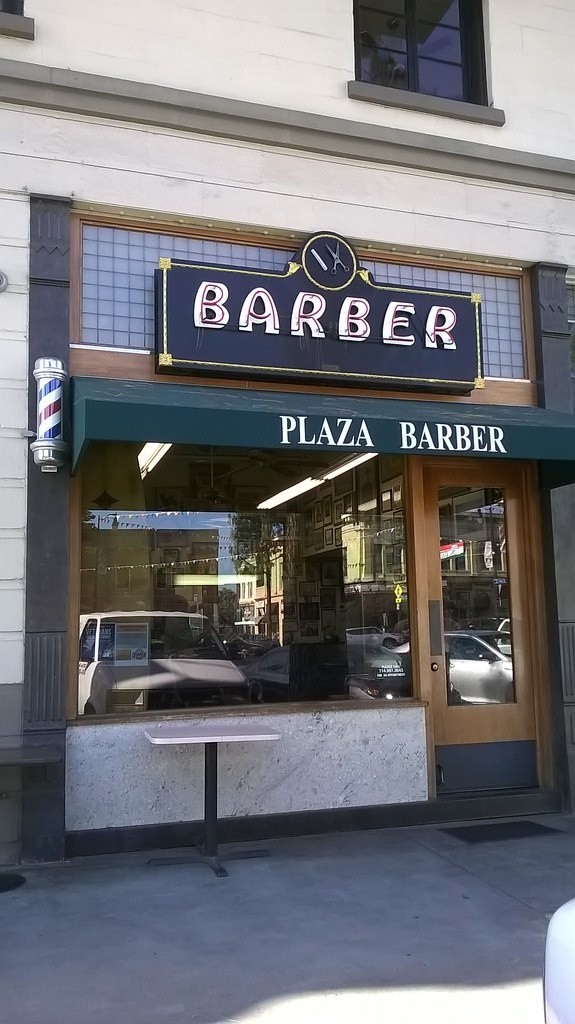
[237,555,271,641]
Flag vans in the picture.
[76,610,250,720]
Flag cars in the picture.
[225,607,516,721]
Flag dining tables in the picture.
[143,723,283,877]
